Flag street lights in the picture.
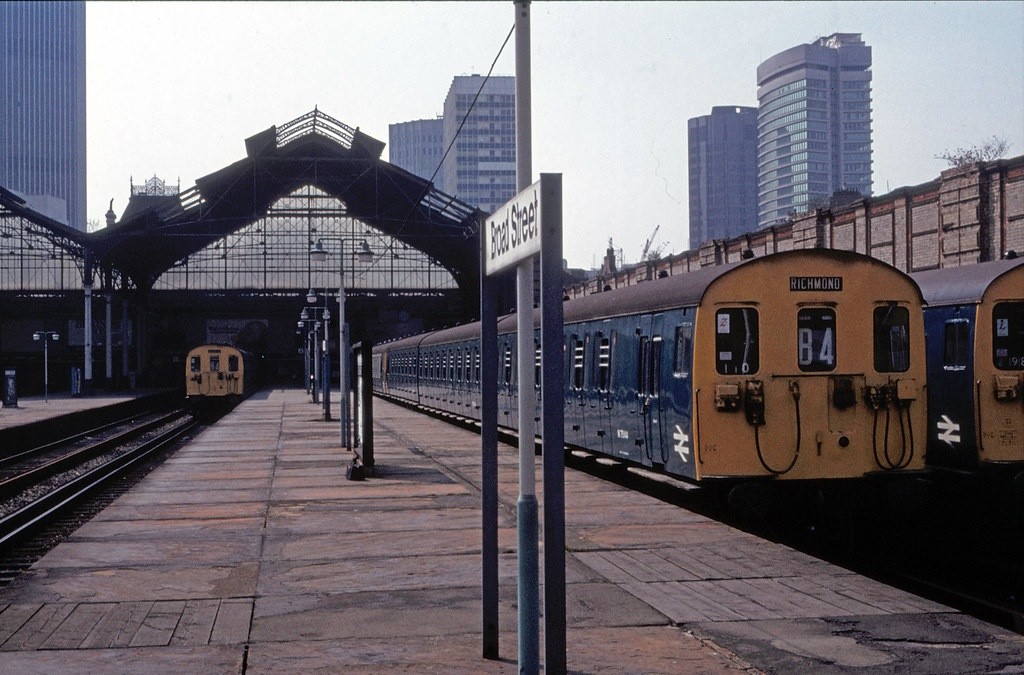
[32,330,59,404]
[297,287,346,421]
[307,236,375,449]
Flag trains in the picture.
[362,245,931,505]
[183,342,265,410]
[902,254,1024,503]
